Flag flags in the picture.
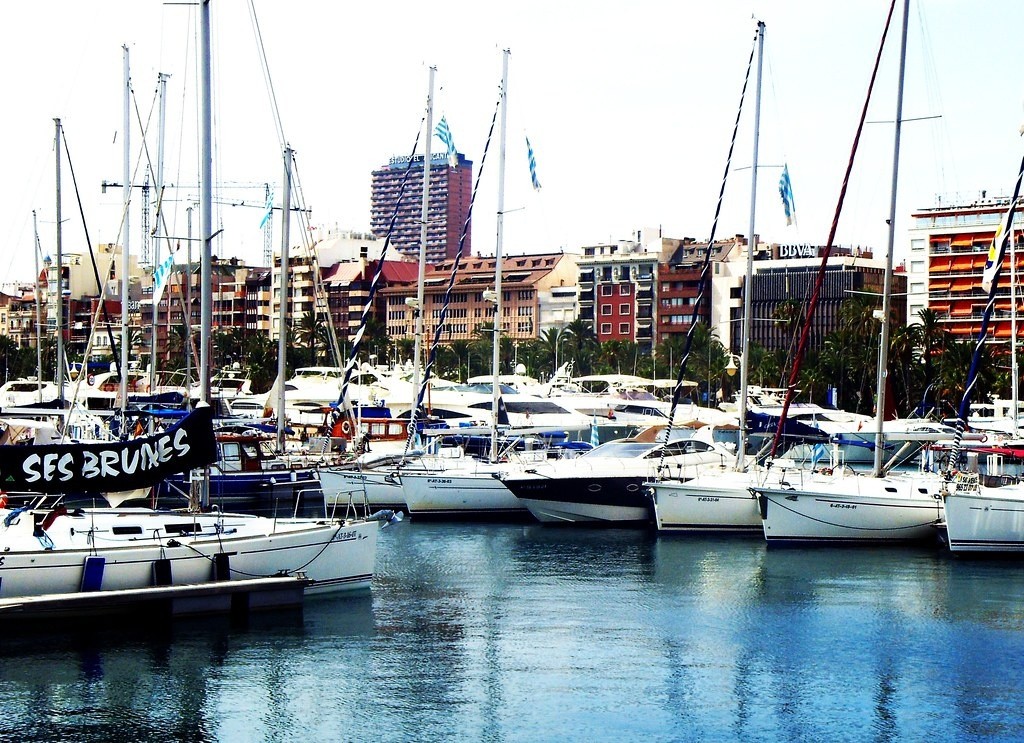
[981,212,1012,293]
[525,136,543,190]
[152,255,173,306]
[779,163,796,226]
[812,423,825,468]
[591,417,599,447]
[432,116,458,169]
[260,192,274,228]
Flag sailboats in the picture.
[1,0,1024,628]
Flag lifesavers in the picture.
[342,421,351,434]
[0,489,8,508]
